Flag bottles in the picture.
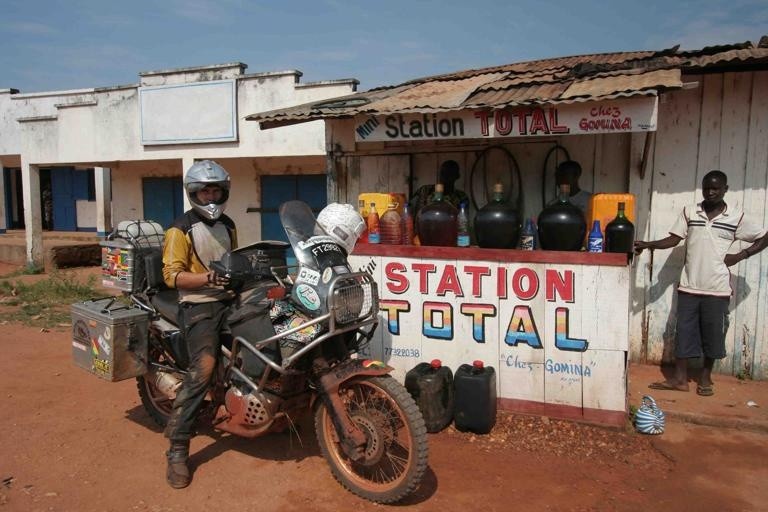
[456,198,475,249]
[537,180,582,250]
[521,215,537,250]
[361,200,380,244]
[401,202,415,244]
[605,200,634,262]
[587,220,605,253]
[472,181,517,246]
[415,181,457,245]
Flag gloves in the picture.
[206,270,230,286]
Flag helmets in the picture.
[184,160,231,221]
[314,201,367,254]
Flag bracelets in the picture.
[743,249,750,257]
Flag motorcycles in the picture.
[60,199,426,507]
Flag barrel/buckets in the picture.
[454,361,497,435]
[404,360,453,433]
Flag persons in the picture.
[410,160,469,235]
[42,183,53,231]
[548,161,592,223]
[634,171,768,396]
[162,160,303,488]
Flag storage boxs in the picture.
[358,192,404,218]
[587,191,636,240]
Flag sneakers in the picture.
[166,448,192,489]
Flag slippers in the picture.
[648,381,690,390]
[697,382,714,396]
